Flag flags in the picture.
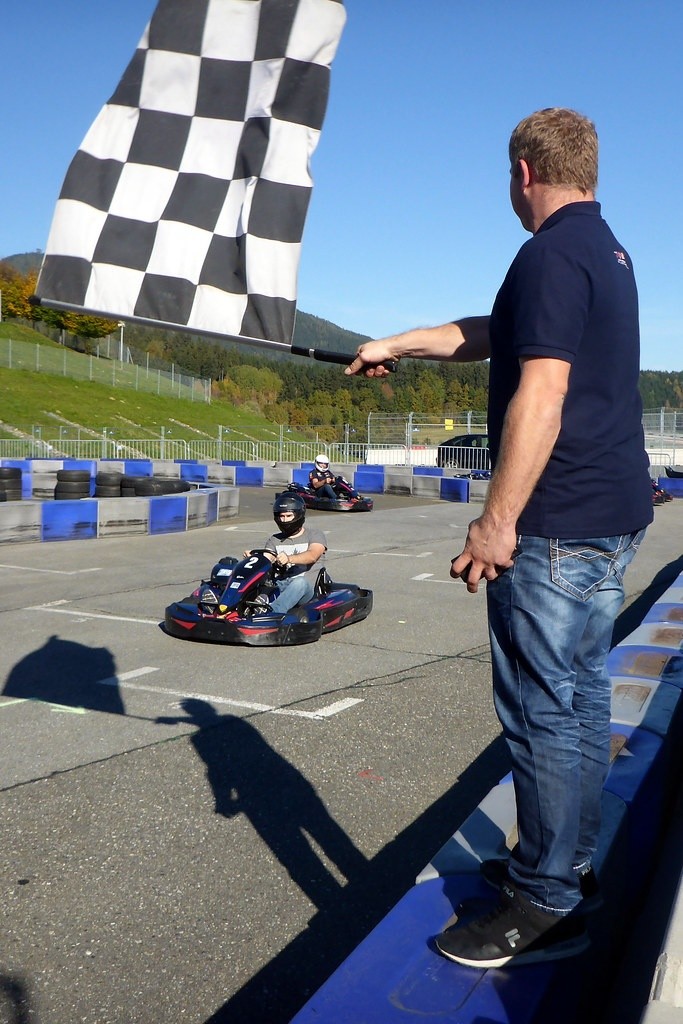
[35,1,347,348]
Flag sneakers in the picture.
[435,880,591,967]
[480,859,597,911]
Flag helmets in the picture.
[273,492,307,536]
[315,455,330,472]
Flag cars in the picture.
[436,433,490,468]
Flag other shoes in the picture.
[242,593,269,621]
[203,589,217,615]
[339,493,344,499]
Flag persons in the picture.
[202,493,328,615]
[309,454,360,502]
[341,109,653,968]
